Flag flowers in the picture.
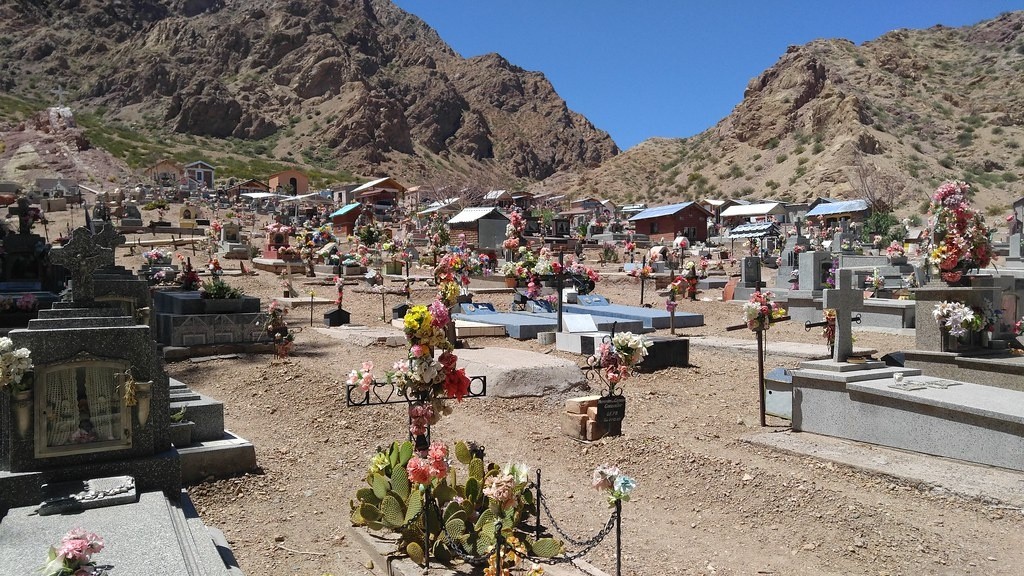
[1,178,1024,575]
